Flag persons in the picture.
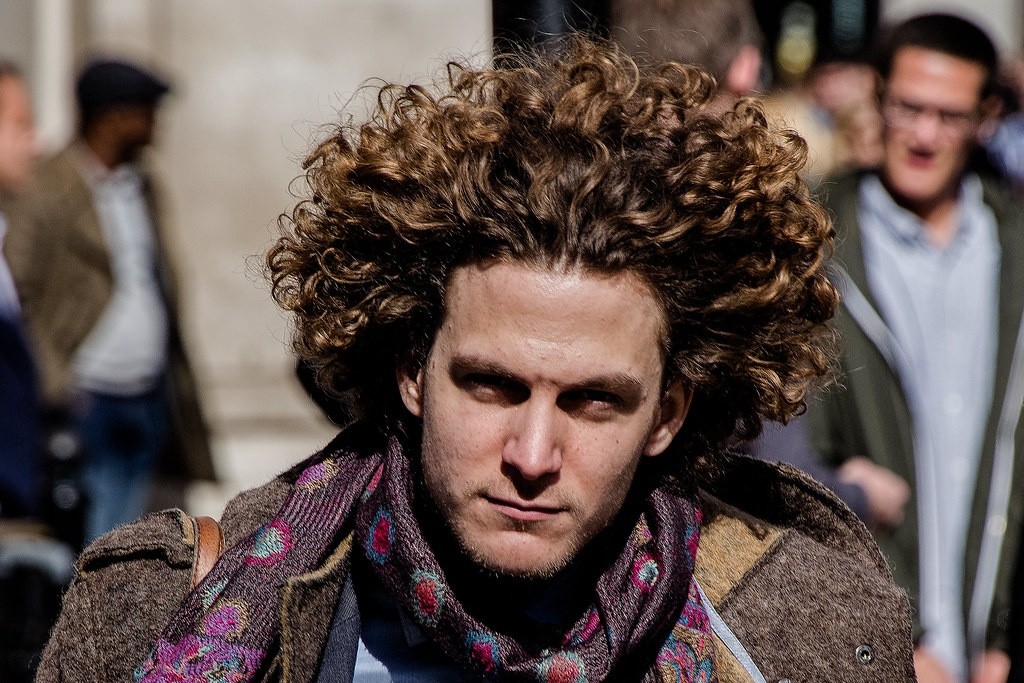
[614,1,1024,683]
[0,59,215,603]
[35,37,917,683]
